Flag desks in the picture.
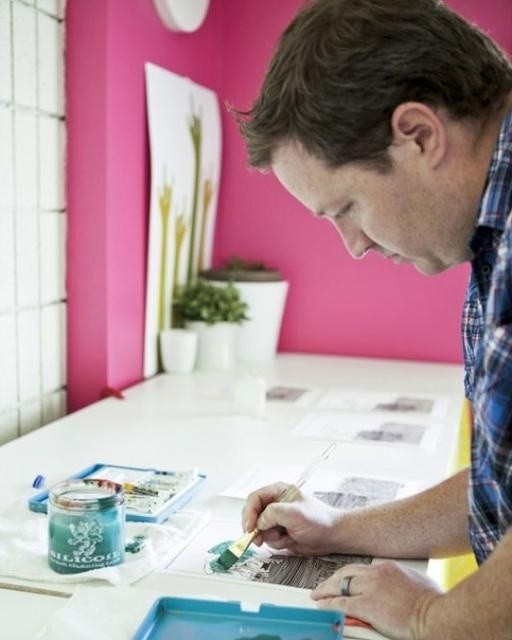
[0,353,478,639]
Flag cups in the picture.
[46,477,126,575]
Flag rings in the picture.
[340,575,352,596]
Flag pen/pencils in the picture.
[122,469,201,514]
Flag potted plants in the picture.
[175,282,250,369]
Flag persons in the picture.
[227,0,511,639]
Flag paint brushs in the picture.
[216,442,338,569]
